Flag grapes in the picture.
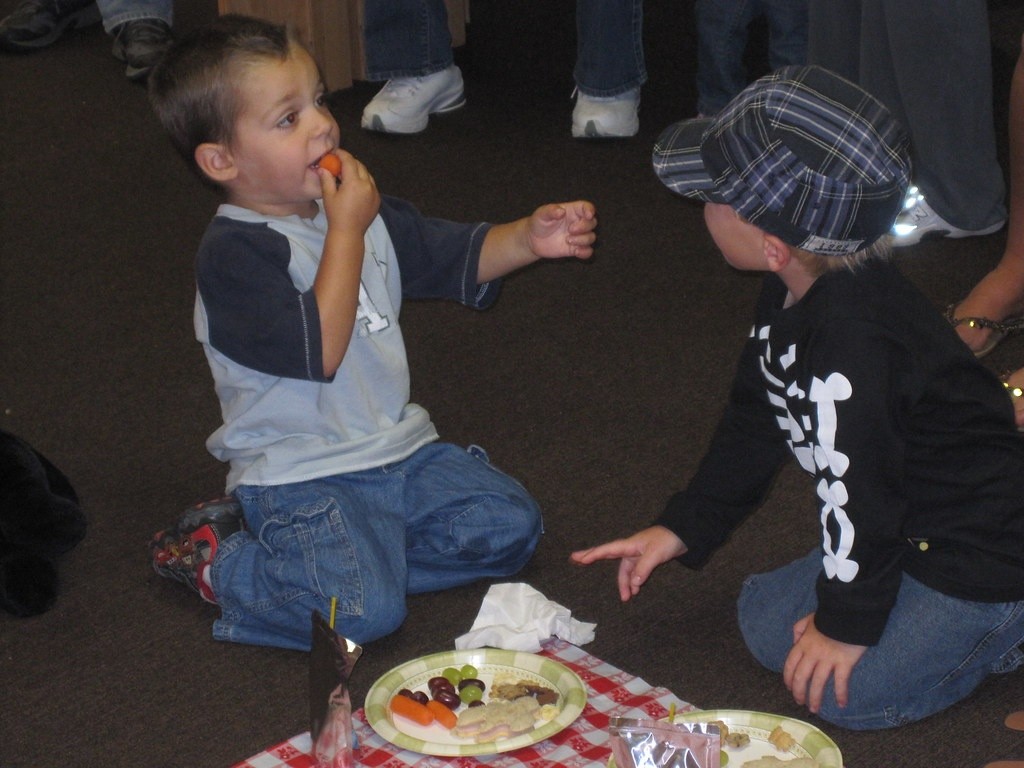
[398,664,486,712]
[720,750,729,766]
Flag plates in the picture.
[366,646,585,756]
[607,709,842,768]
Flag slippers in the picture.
[1001,369,1024,431]
[945,304,1024,357]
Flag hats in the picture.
[652,65,911,255]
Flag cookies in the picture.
[455,674,560,743]
[706,720,820,768]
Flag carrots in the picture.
[317,153,341,176]
[388,694,434,726]
[426,699,458,729]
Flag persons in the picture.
[941,46,1023,431]
[357,0,647,140]
[692,0,1009,248]
[144,11,598,653]
[0,0,177,80]
[567,65,1024,730]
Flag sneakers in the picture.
[112,19,178,79]
[362,64,466,133]
[569,86,641,139]
[890,186,1007,245]
[143,497,243,595]
[0,0,102,48]
